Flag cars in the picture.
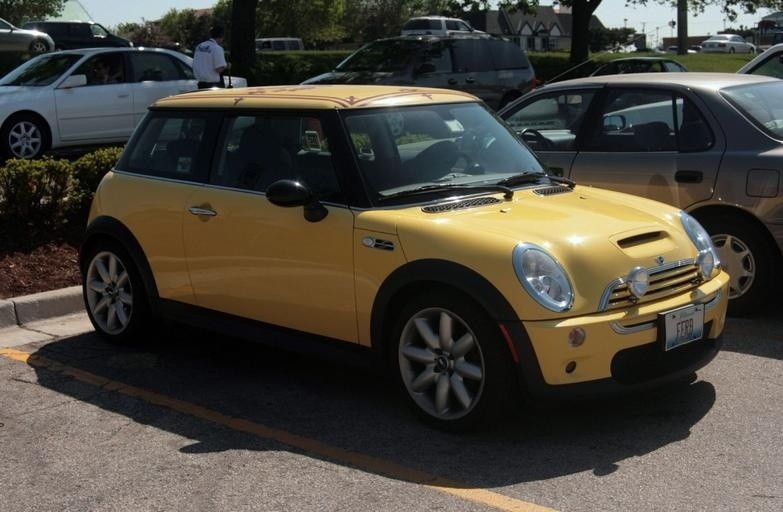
[736,41,783,79]
[590,58,688,77]
[702,33,758,54]
[0,17,54,56]
[495,72,783,318]
[78,81,729,433]
[0,46,255,161]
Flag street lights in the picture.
[655,25,662,51]
[641,20,646,34]
[723,17,727,31]
[622,18,628,27]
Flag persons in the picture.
[192,27,231,88]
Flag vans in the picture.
[23,20,132,52]
[255,36,303,52]
[298,35,538,110]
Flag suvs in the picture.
[401,14,484,36]
